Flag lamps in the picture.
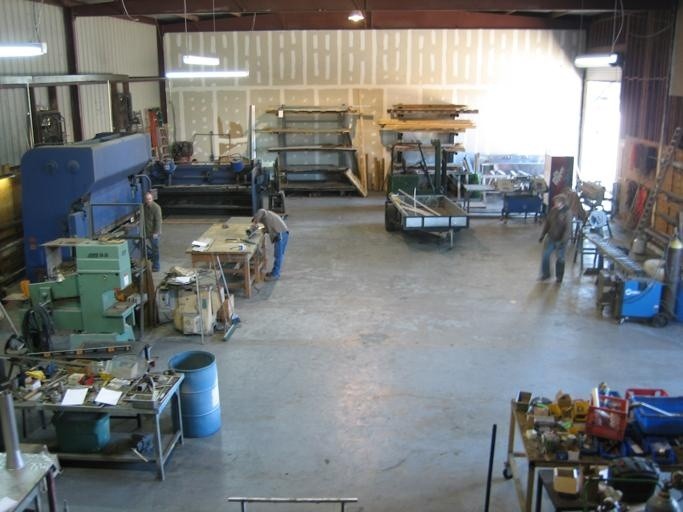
[153,11,256,79]
[0,0,48,58]
[347,9,365,23]
[181,0,220,66]
[572,0,617,67]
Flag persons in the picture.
[251,208,290,280]
[537,193,571,285]
[141,191,162,273]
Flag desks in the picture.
[185,221,266,300]
[12,371,187,484]
[501,399,682,512]
[0,451,62,512]
[534,469,604,511]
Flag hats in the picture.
[551,193,570,204]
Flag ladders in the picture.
[630,127,683,246]
[148,107,171,161]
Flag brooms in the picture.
[216,255,241,325]
[210,253,236,341]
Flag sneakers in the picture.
[263,270,282,284]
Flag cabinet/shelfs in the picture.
[264,103,362,197]
